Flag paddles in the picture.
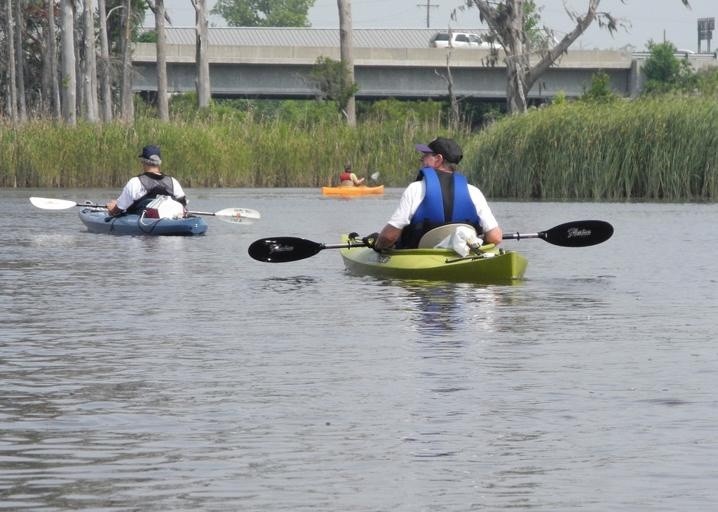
[28,196,260,225]
[365,171,380,181]
[247,220,613,263]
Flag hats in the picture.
[415,137,463,164]
[139,145,161,161]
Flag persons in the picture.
[374,138,503,253]
[106,145,188,218]
[339,161,365,186]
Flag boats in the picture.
[79,198,208,238]
[339,231,529,285]
[320,184,385,196]
